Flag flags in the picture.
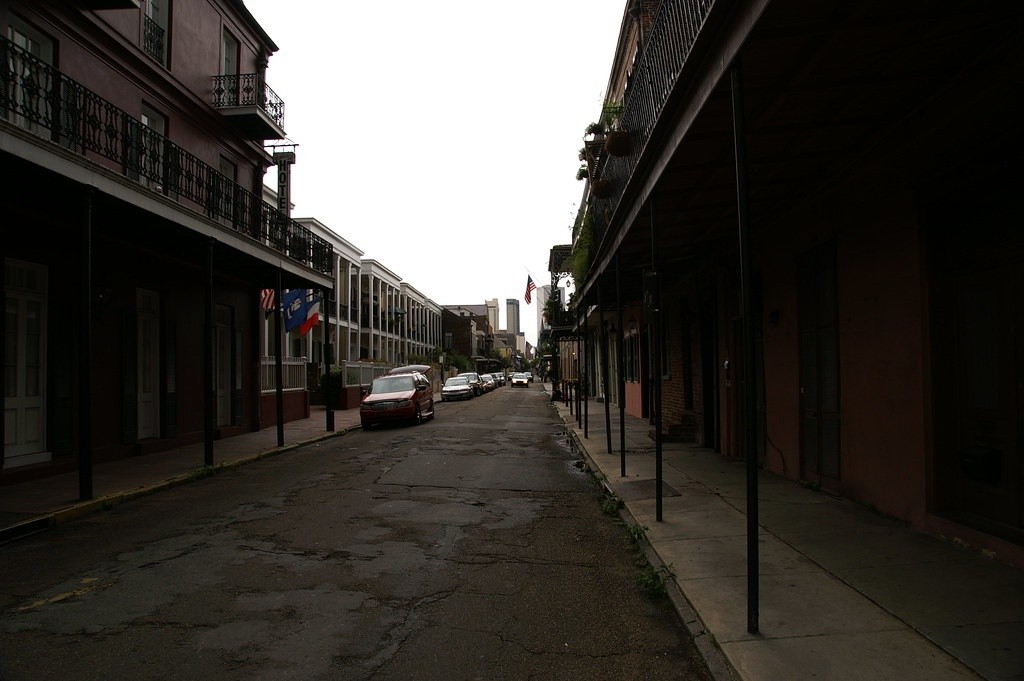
[300,295,320,337]
[282,287,308,334]
[524,274,537,305]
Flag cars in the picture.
[458,372,505,397]
[360,364,435,430]
[508,371,534,382]
[511,373,528,387]
[441,377,473,402]
